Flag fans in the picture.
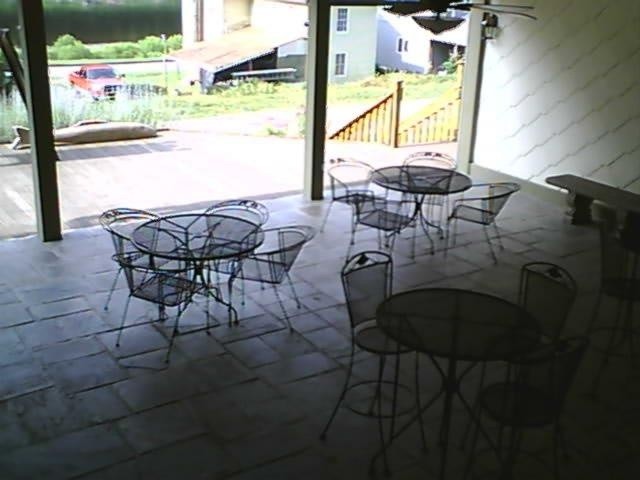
[320,1,539,34]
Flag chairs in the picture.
[596,216,639,368]
[94,197,319,366]
[321,149,523,268]
[321,249,602,478]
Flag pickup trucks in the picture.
[67,63,127,103]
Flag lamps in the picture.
[479,11,502,40]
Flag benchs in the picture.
[543,165,640,246]
